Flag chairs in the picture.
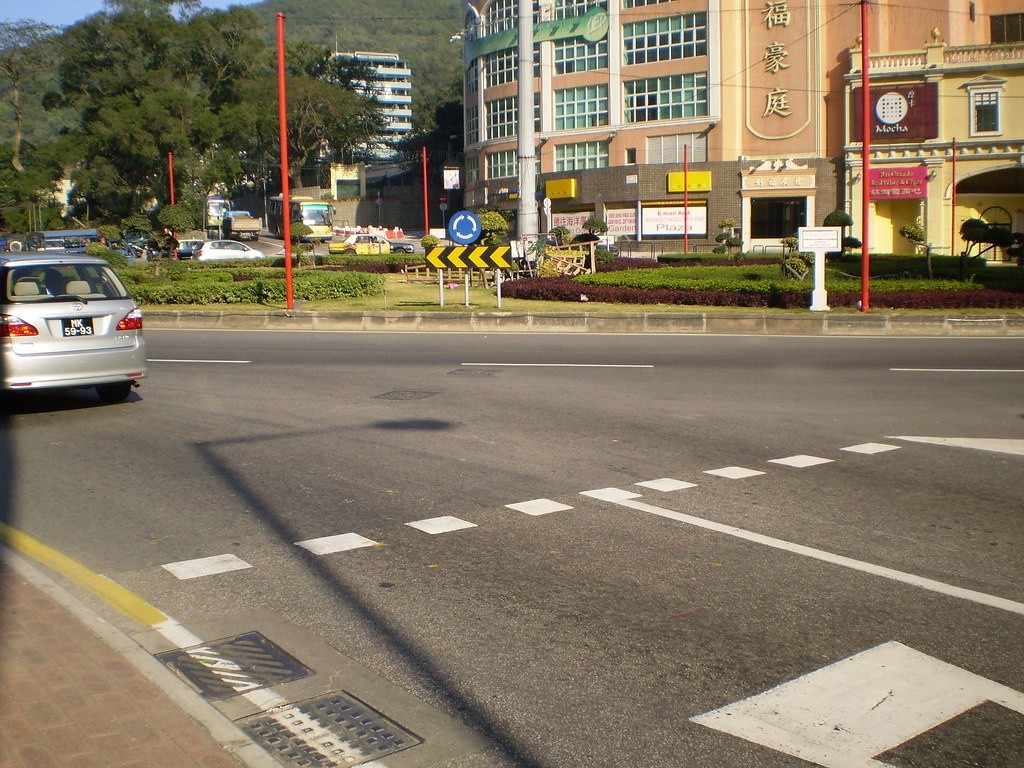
[66,282,91,295]
[14,282,39,297]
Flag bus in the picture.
[267,193,336,243]
[206,198,233,229]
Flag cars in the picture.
[596,244,622,257]
[328,233,415,257]
[0,249,149,410]
[101,226,204,267]
[194,239,267,260]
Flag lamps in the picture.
[856,172,862,181]
[709,121,718,127]
[475,146,483,151]
[930,169,937,178]
[541,137,549,142]
[609,130,617,139]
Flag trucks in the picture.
[221,211,263,242]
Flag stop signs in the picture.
[439,194,448,203]
